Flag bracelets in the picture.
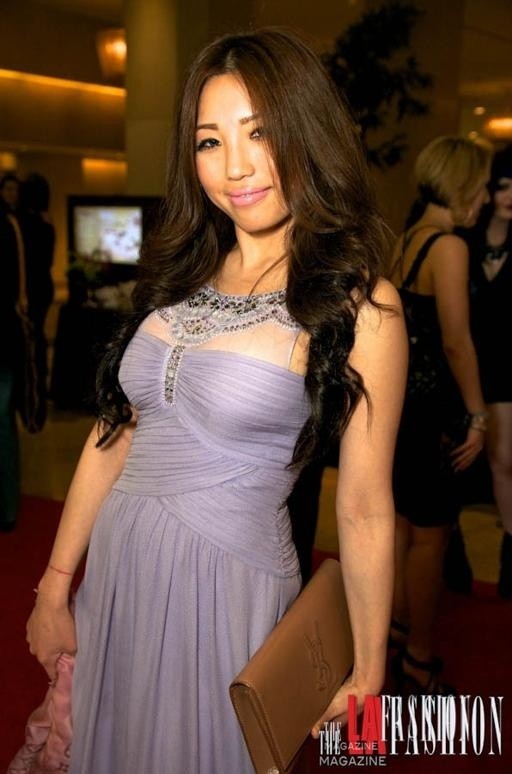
[466,412,489,431]
[47,564,71,578]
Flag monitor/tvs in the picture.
[66,194,163,273]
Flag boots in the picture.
[441,525,472,597]
[498,532,512,599]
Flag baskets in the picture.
[440,433,465,480]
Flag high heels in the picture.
[388,620,454,704]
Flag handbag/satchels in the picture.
[228,558,355,774]
[16,306,47,431]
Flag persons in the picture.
[439,144,512,601]
[386,132,494,702]
[24,28,413,773]
[0,162,58,535]
